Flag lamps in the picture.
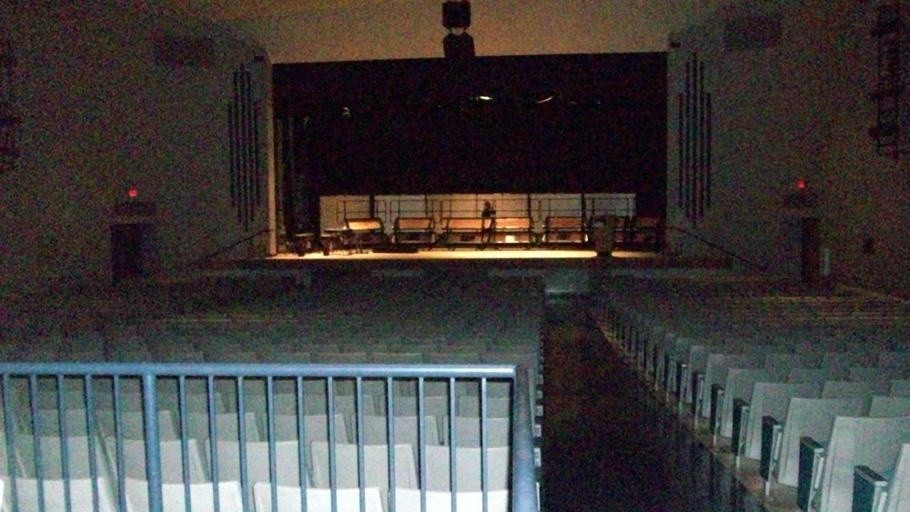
[441,0,479,87]
